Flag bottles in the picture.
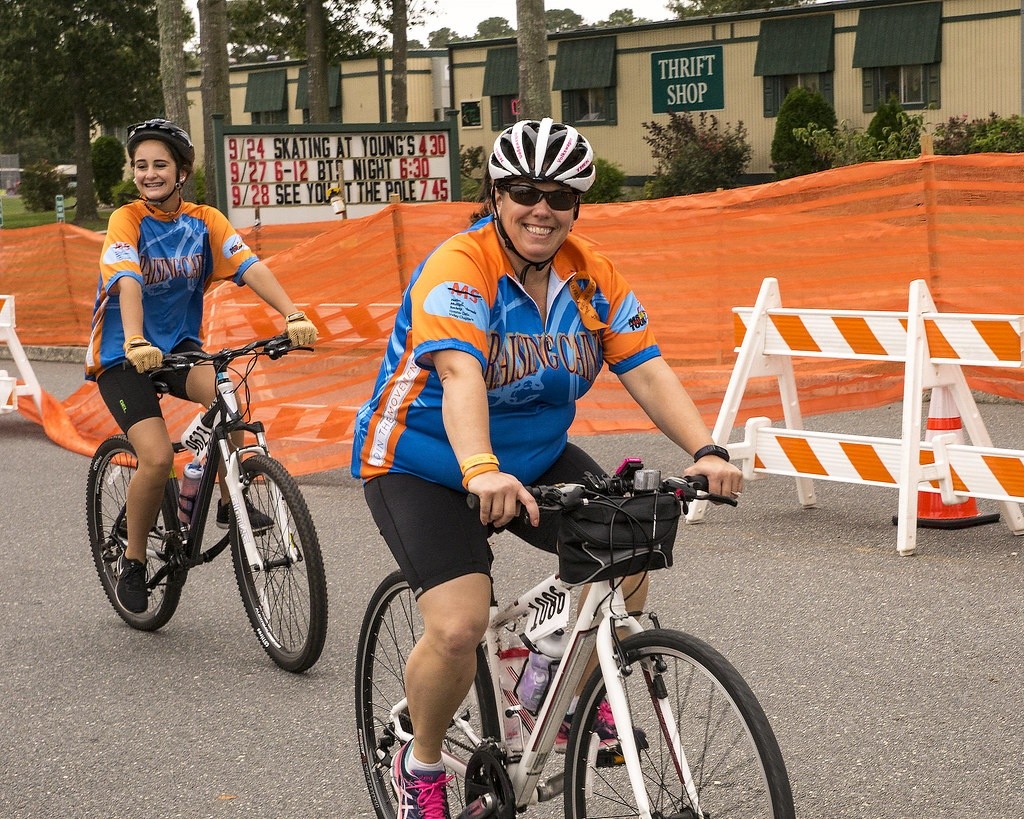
[515,627,565,712]
[498,637,537,752]
[177,457,204,524]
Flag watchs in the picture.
[693,444,730,463]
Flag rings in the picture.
[732,490,741,497]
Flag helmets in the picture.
[487,117,596,194]
[126,117,196,180]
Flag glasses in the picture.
[499,183,581,211]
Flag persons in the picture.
[84,120,318,615]
[350,122,745,819]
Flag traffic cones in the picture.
[893,385,1001,530]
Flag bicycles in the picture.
[85,334,329,672]
[352,461,797,819]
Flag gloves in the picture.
[123,335,163,374]
[286,312,318,349]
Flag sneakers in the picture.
[551,699,648,752]
[388,738,451,819]
[216,500,276,533]
[115,552,148,613]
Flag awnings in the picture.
[552,36,617,91]
[851,2,942,68]
[753,13,834,76]
[243,69,287,112]
[295,65,342,109]
[482,48,518,95]
